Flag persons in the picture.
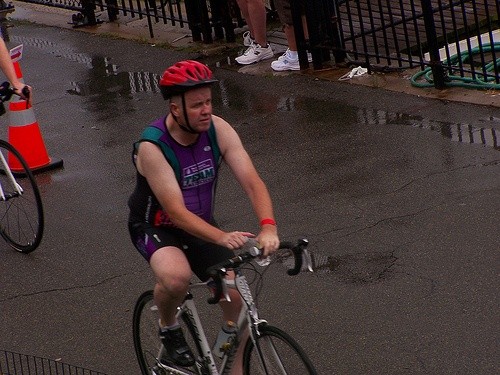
[270,0,312,71]
[232,0,274,64]
[127,60,280,375]
[0,37,33,104]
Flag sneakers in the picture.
[269,48,313,72]
[234,42,273,65]
[158,325,195,367]
[243,30,254,46]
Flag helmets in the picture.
[157,60,220,101]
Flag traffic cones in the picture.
[0,62,63,176]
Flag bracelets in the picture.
[258,217,278,227]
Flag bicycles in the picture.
[0,81,44,254]
[132,238,317,375]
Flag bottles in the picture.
[212,321,237,358]
